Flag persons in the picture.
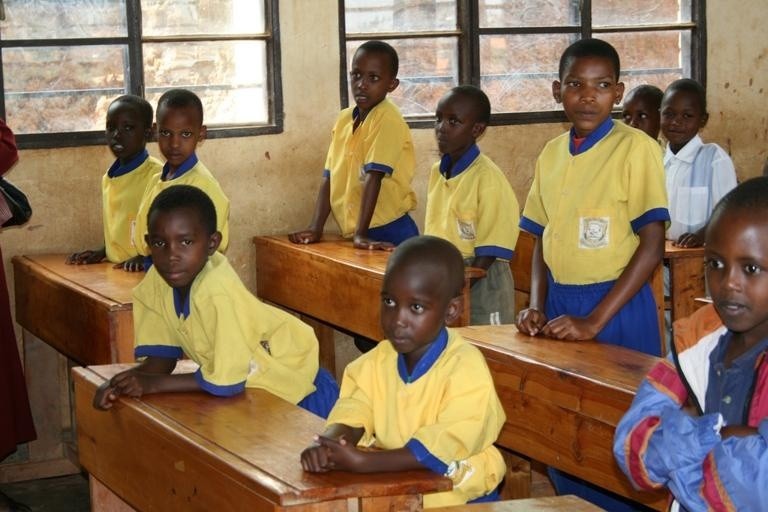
[660,78,738,248]
[300,235,506,509]
[94,184,340,420]
[423,84,520,326]
[512,39,671,358]
[66,94,164,272]
[0,119,38,512]
[133,88,231,272]
[622,84,666,160]
[288,41,420,353]
[614,177,768,512]
